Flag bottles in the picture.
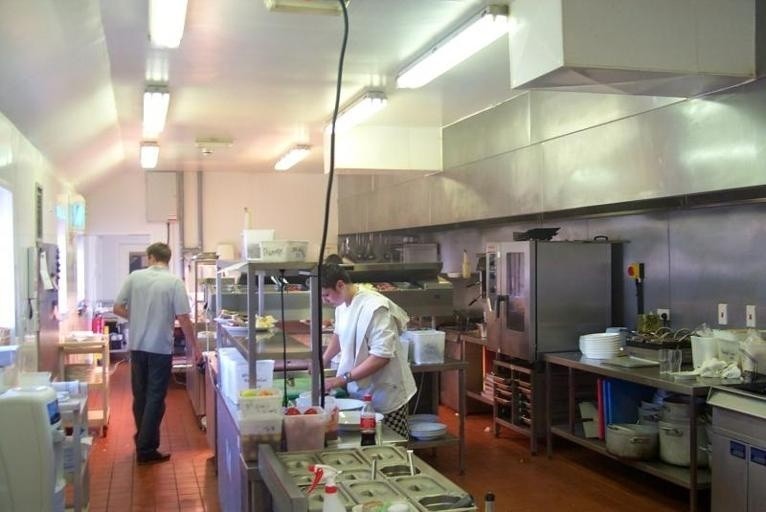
[360,395,376,448]
[463,249,471,279]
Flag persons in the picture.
[319,263,418,439]
[112,243,207,464]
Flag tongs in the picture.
[369,450,415,480]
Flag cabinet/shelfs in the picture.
[441,341,491,413]
[711,405,766,512]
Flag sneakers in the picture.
[137,450,170,465]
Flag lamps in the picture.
[141,142,161,168]
[148,0,188,48]
[276,144,311,171]
[143,85,170,134]
[395,5,508,88]
[323,88,389,134]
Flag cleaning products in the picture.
[305,464,345,512]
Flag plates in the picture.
[668,372,700,379]
[579,333,626,360]
[328,398,384,432]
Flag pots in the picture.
[606,397,701,467]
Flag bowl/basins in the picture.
[408,414,449,440]
[448,273,463,278]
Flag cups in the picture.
[658,348,682,375]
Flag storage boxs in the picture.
[217,229,308,262]
[218,349,274,405]
[403,243,437,263]
[400,330,446,364]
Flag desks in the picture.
[58,394,91,512]
[59,334,109,438]
[101,319,128,353]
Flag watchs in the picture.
[343,372,351,383]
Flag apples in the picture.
[285,408,317,415]
